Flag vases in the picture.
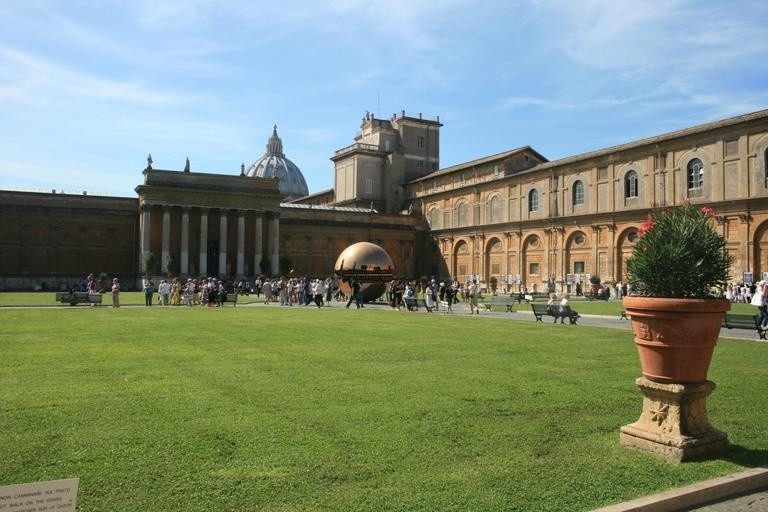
[623,296,731,384]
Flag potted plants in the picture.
[489,276,498,290]
[143,252,157,277]
[167,255,177,277]
[420,275,429,289]
[590,275,601,294]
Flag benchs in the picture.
[199,294,237,307]
[57,292,103,303]
[530,304,581,325]
[404,297,436,312]
[477,292,525,312]
[584,292,603,301]
[721,313,766,341]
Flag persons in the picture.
[142,274,154,307]
[560,294,575,324]
[357,280,365,308]
[546,293,563,324]
[157,276,335,308]
[385,277,480,314]
[709,280,767,333]
[604,280,640,299]
[344,277,360,308]
[87,271,97,307]
[576,282,581,296]
[112,276,122,308]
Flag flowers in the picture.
[622,196,735,300]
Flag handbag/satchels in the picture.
[750,292,765,307]
